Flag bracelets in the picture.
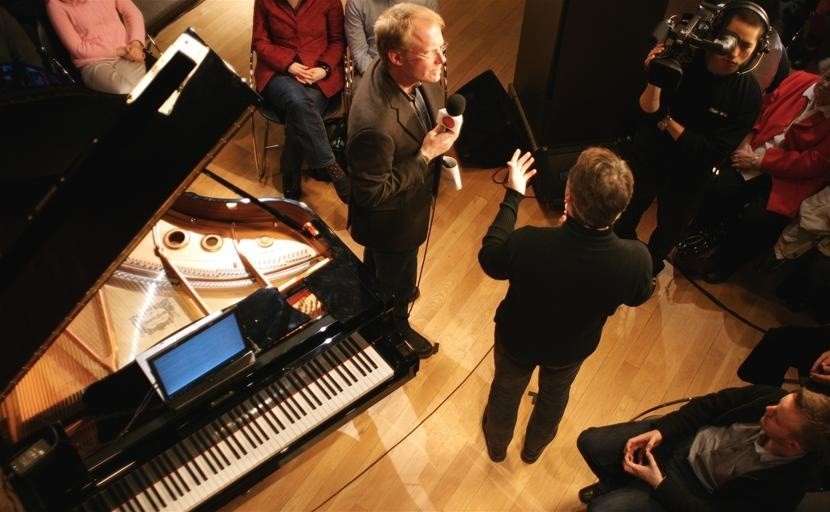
[751,154,761,167]
[419,148,433,165]
[321,64,331,79]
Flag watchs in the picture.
[658,116,672,131]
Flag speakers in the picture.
[454,69,531,169]
[536,146,585,210]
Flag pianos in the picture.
[0,28,420,510]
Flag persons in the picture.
[616,2,771,291]
[343,0,444,102]
[686,57,829,283]
[477,145,655,465]
[250,1,356,205]
[578,387,829,510]
[680,325,830,428]
[781,2,828,69]
[345,3,458,359]
[774,185,829,312]
[45,0,150,99]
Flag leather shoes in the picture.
[398,327,434,358]
[283,169,351,205]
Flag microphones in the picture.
[432,94,466,159]
[736,52,764,78]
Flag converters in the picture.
[8,439,53,477]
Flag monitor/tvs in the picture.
[146,304,247,410]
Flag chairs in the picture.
[629,398,806,512]
[345,43,448,113]
[35,0,162,89]
[248,52,351,183]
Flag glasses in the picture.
[405,41,448,61]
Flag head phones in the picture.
[732,0,772,53]
